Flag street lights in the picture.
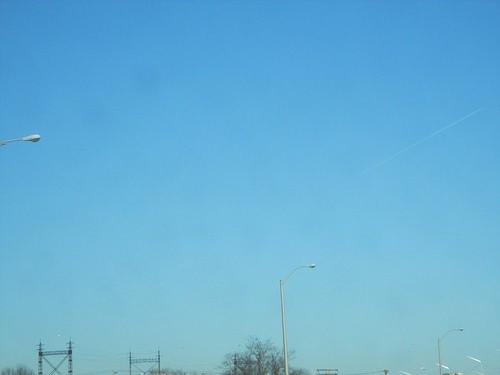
[279,263,317,375]
[437,327,463,375]
